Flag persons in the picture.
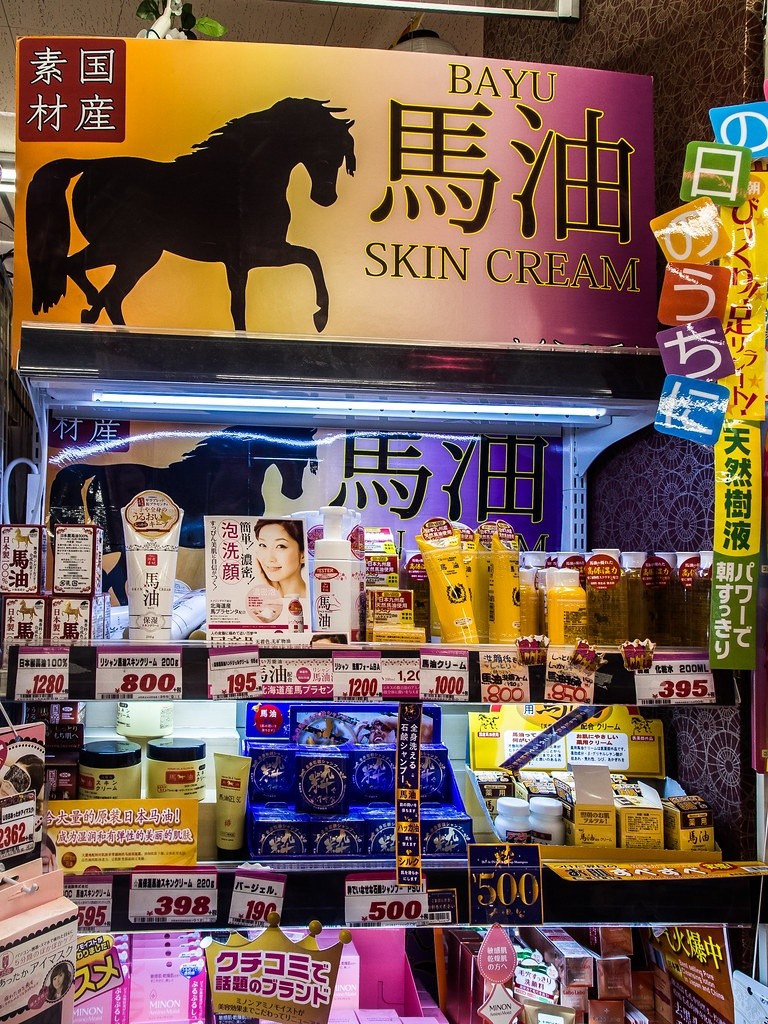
[354,713,434,744]
[48,963,70,999]
[314,731,325,744]
[41,831,57,874]
[0,755,44,804]
[252,520,308,597]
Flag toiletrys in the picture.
[0,470,768,1024]
